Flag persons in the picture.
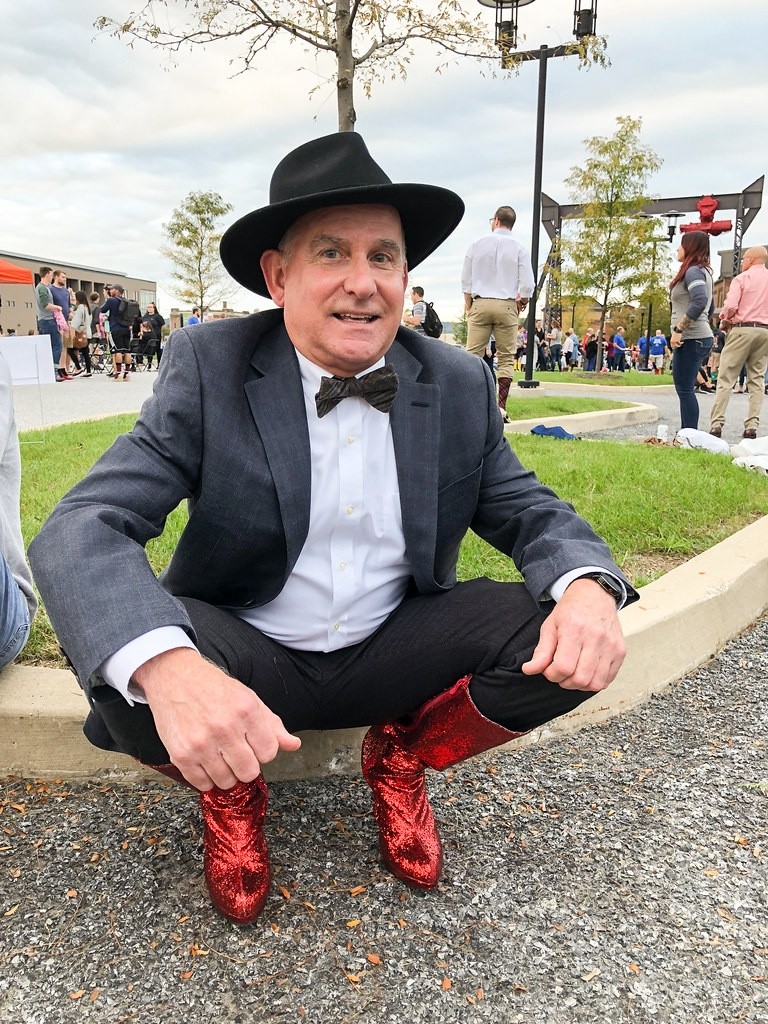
[513,320,579,372]
[88,291,101,338]
[708,246,768,438]
[67,287,92,377]
[207,314,213,321]
[696,319,768,394]
[50,269,73,380]
[188,308,201,324]
[34,267,65,382]
[669,231,713,430]
[24,133,641,922]
[130,300,165,372]
[101,284,131,381]
[482,334,496,385]
[0,348,38,674]
[460,206,535,424]
[578,328,606,371]
[605,326,672,374]
[403,286,426,337]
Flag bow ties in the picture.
[314,363,401,418]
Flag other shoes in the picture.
[52,359,162,386]
[498,406,512,423]
[743,429,757,439]
[514,358,768,403]
[709,427,722,437]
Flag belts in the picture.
[733,322,768,329]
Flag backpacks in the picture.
[419,301,443,339]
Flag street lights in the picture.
[479,0,598,389]
[632,209,686,371]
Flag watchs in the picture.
[581,572,623,604]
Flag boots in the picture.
[361,675,536,890]
[130,757,271,926]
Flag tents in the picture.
[0,259,35,292]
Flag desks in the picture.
[0,334,56,385]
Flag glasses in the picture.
[489,218,496,225]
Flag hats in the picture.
[219,132,466,299]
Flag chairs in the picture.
[70,337,159,372]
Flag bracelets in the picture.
[674,326,682,333]
[520,301,528,305]
